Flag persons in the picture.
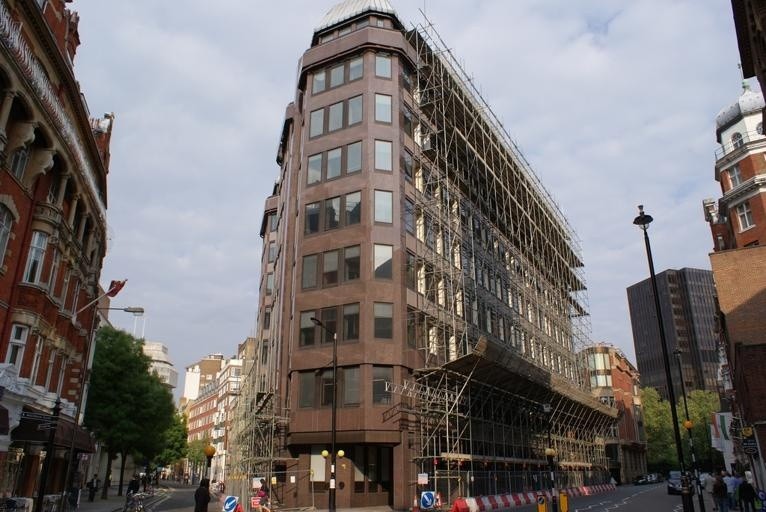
[0,488,18,512]
[703,467,760,512]
[141,476,146,492]
[86,474,98,503]
[257,478,270,512]
[121,472,139,512]
[193,478,212,512]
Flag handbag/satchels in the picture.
[257,490,266,497]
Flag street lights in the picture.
[545,448,557,511]
[147,359,174,366]
[310,316,345,512]
[63,304,147,512]
[632,204,696,511]
[672,343,707,512]
[202,441,216,493]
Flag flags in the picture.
[104,278,126,299]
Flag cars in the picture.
[666,469,705,498]
[635,473,664,484]
